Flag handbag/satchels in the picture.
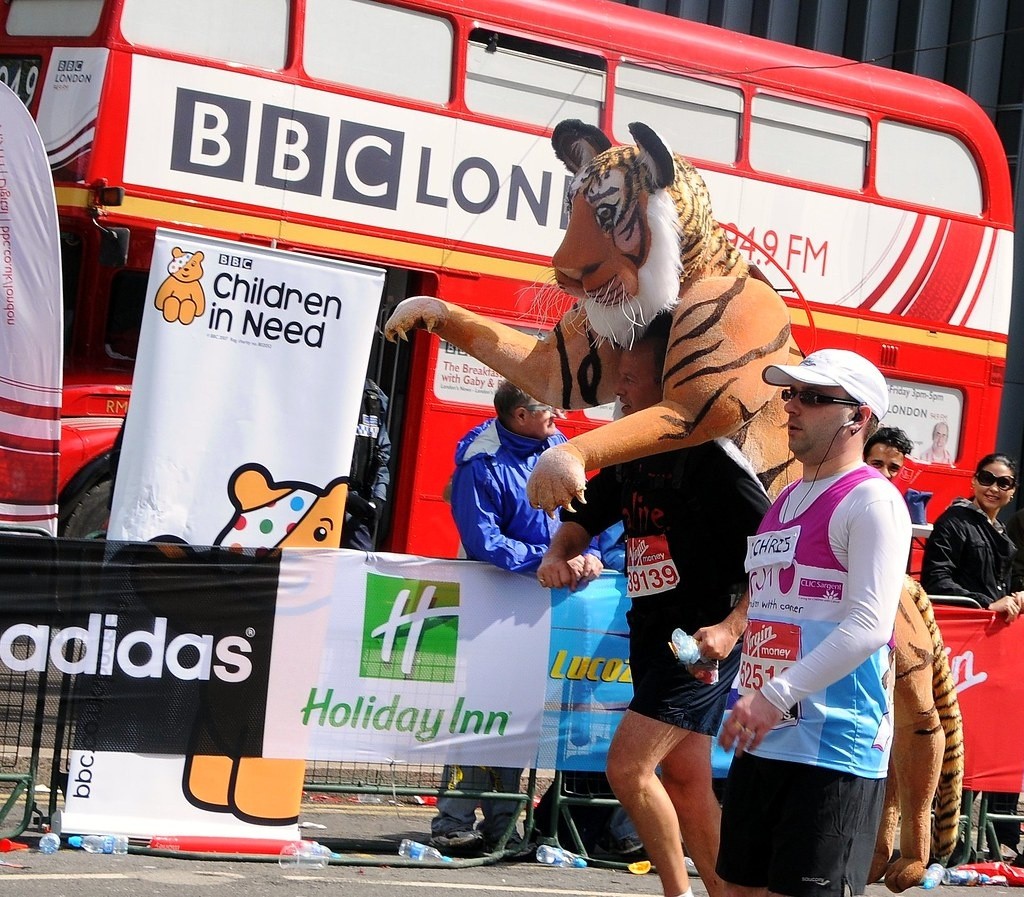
[904,487,933,526]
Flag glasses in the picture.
[781,389,861,407]
[974,471,1018,492]
[525,403,553,412]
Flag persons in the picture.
[536,309,773,897]
[715,347,913,897]
[429,379,604,858]
[919,422,953,463]
[863,427,915,576]
[920,452,1024,868]
[522,519,644,854]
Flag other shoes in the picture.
[428,828,485,852]
[482,833,539,862]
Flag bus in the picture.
[0,0,1016,580]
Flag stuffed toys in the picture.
[383,118,964,894]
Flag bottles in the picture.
[397,838,451,862]
[68,835,129,855]
[39,832,60,856]
[923,863,946,889]
[941,869,990,886]
[536,844,587,869]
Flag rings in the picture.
[538,577,545,582]
[733,720,743,730]
[741,727,752,736]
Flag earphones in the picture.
[842,413,863,427]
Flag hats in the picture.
[762,348,889,423]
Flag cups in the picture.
[278,845,330,870]
